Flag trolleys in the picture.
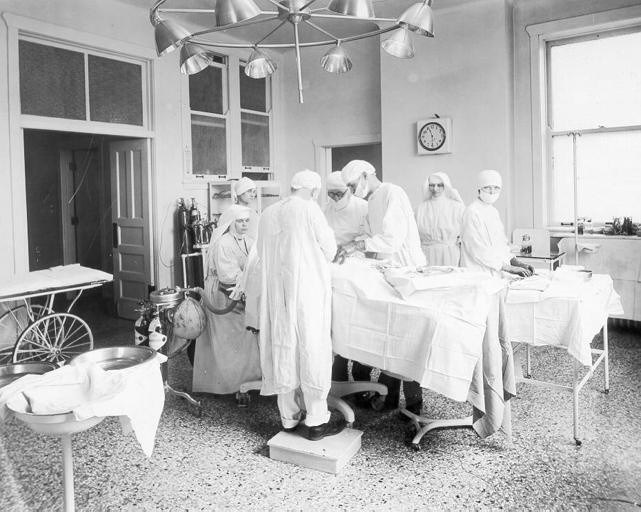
[0,264,114,363]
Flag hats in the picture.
[326,171,344,191]
[342,159,377,184]
[234,176,257,195]
[291,170,321,188]
[476,169,503,189]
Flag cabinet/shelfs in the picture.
[501,268,626,448]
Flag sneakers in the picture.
[380,403,398,413]
[402,405,420,419]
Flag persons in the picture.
[414,171,464,267]
[191,204,256,396]
[460,170,535,278]
[337,159,427,422]
[234,177,257,205]
[321,171,368,410]
[256,170,346,441]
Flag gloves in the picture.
[334,241,366,265]
[479,190,499,204]
[504,256,535,277]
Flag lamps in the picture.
[152,1,435,80]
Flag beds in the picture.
[234,261,505,450]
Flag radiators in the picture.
[560,239,641,328]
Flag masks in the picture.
[352,178,369,198]
[328,192,348,210]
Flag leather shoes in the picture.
[357,391,370,401]
[283,409,306,432]
[311,410,345,440]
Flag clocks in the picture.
[416,117,451,156]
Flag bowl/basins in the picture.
[0,345,156,436]
